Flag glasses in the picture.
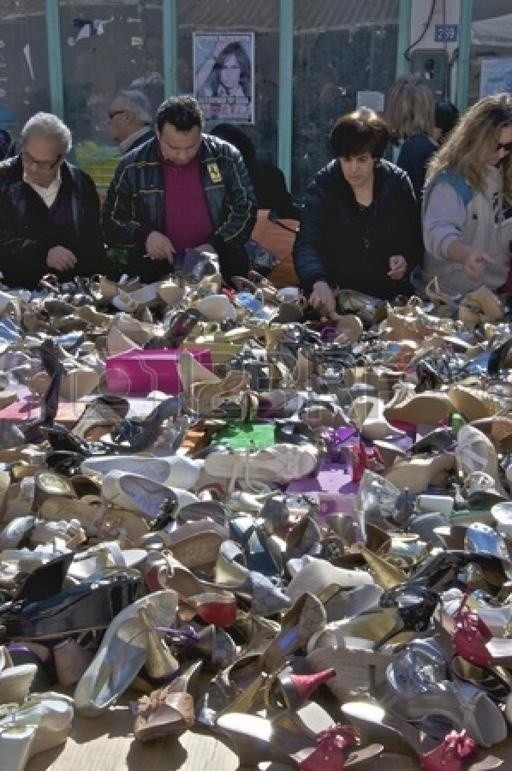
[488,141,511,153]
[19,147,61,171]
[109,109,124,119]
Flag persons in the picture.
[208,122,299,220]
[108,89,155,154]
[383,76,442,206]
[431,99,458,145]
[204,40,252,122]
[0,109,108,289]
[409,92,511,302]
[101,94,258,277]
[290,113,421,319]
